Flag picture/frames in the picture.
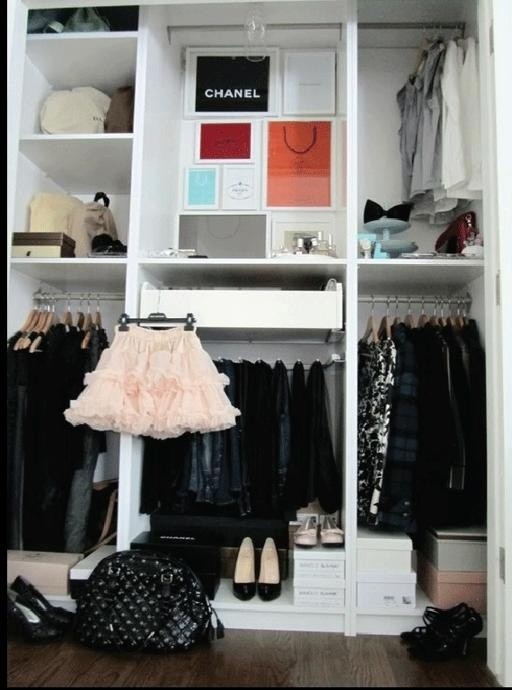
[174,44,346,261]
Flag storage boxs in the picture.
[9,231,76,259]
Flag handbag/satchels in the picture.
[28,191,117,258]
[82,479,118,556]
[434,212,476,253]
[76,549,224,654]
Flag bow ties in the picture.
[363,199,412,223]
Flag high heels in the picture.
[400,603,483,659]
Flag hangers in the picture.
[359,290,470,348]
[412,22,433,76]
[16,293,104,352]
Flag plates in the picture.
[367,240,418,254]
[362,219,412,233]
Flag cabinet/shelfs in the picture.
[3,1,487,636]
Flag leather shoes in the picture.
[6,576,75,643]
[320,515,344,547]
[294,515,317,548]
[233,536,256,600]
[257,537,281,601]
